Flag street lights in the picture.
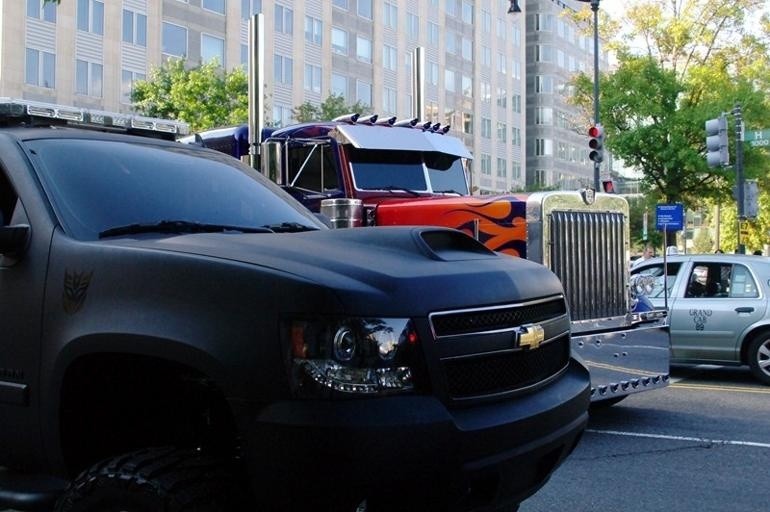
[506,0,600,191]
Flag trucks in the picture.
[179,113,668,402]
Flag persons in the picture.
[634,248,654,266]
[752,250,762,255]
[715,249,730,291]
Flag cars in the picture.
[631,252,769,382]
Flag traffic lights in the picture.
[705,117,731,168]
[586,123,606,166]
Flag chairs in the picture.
[687,278,723,301]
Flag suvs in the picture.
[0,98,590,511]
